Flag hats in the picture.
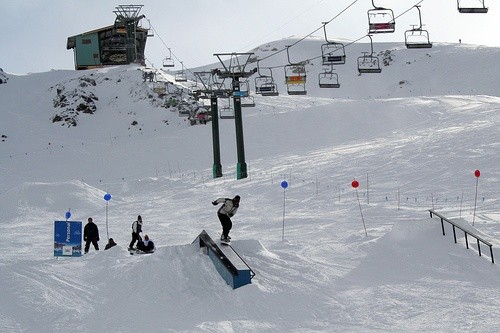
[234,195,240,200]
[138,215,142,219]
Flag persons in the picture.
[104,237,117,250]
[139,235,154,254]
[211,194,240,243]
[128,215,143,250]
[83,217,100,254]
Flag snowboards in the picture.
[221,235,232,245]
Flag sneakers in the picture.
[221,238,230,242]
[221,235,231,239]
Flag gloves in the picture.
[229,212,234,216]
[212,200,218,204]
